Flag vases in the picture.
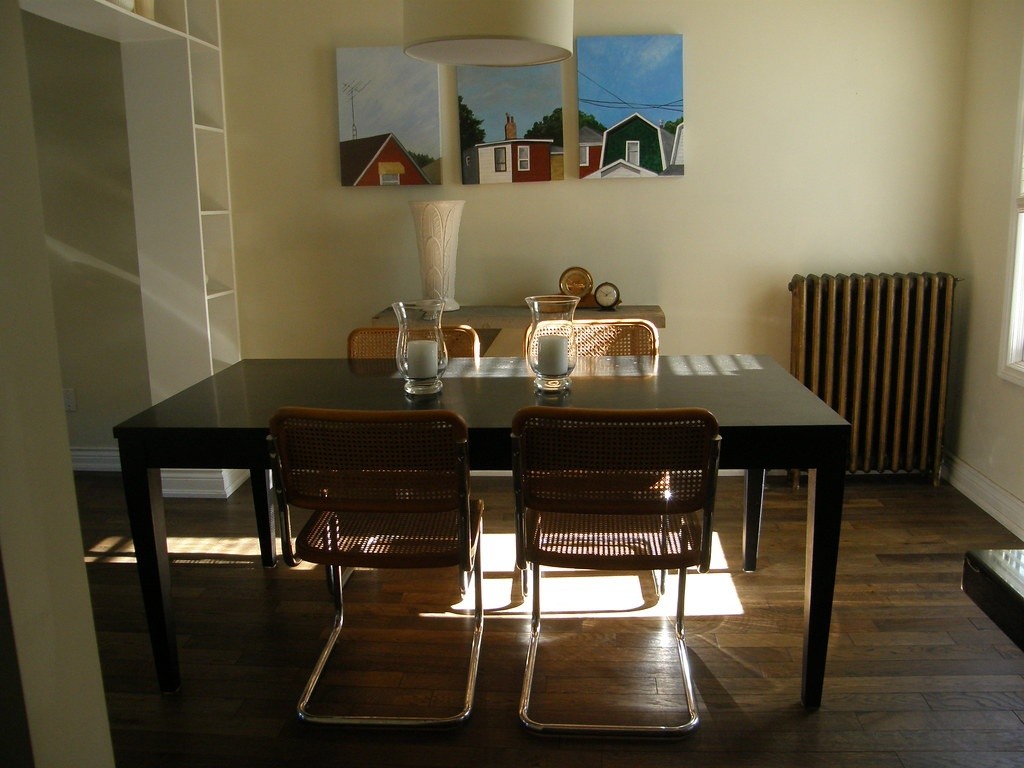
[408,200,466,310]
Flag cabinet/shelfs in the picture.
[21,1,252,499]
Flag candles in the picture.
[407,340,438,378]
[538,336,568,376]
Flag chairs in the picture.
[524,318,660,359]
[510,406,723,736]
[268,405,484,726]
[347,326,482,359]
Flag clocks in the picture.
[595,282,620,312]
[560,268,594,307]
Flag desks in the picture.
[112,359,850,710]
[372,304,666,358]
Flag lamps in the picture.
[403,0,573,67]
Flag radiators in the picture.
[785,271,955,486]
